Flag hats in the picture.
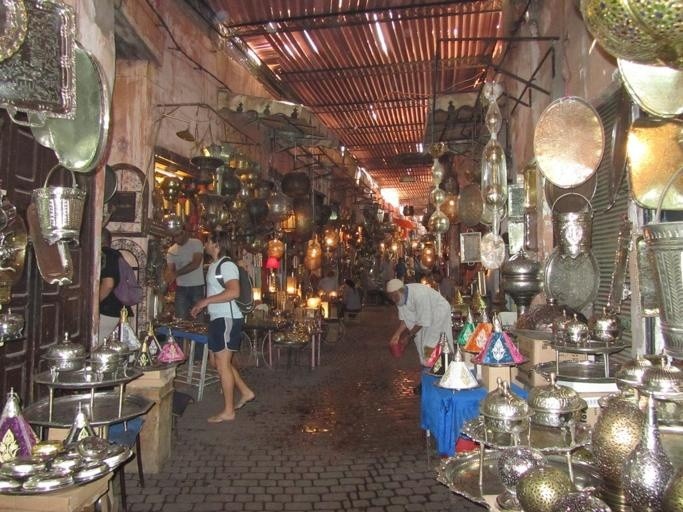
[386,277,404,292]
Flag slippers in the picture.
[234,398,257,411]
[206,416,235,425]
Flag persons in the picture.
[395,257,407,284]
[99,227,134,347]
[190,231,255,424]
[337,279,362,323]
[378,253,394,305]
[163,227,205,362]
[386,279,454,395]
[317,271,340,291]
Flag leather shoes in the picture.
[413,383,422,395]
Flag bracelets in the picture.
[408,332,416,339]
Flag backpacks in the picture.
[215,257,255,314]
[112,253,144,305]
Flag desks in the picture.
[157,325,223,400]
[422,368,529,466]
